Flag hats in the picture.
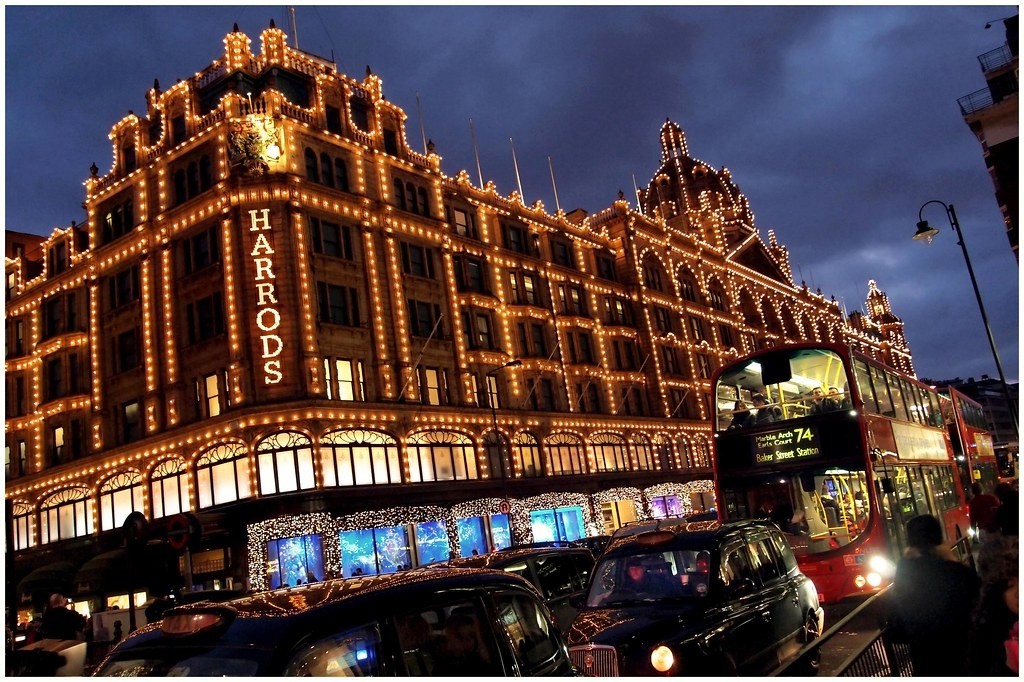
[906,514,945,548]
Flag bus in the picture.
[711,343,973,604]
[934,386,1002,507]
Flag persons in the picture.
[471,550,478,555]
[400,616,434,646]
[695,552,710,572]
[352,568,362,576]
[449,551,455,558]
[146,595,173,623]
[821,485,867,514]
[397,564,410,571]
[626,557,647,580]
[882,486,907,508]
[276,572,318,589]
[751,393,777,423]
[42,594,86,640]
[444,607,492,663]
[809,382,851,412]
[888,482,1019,677]
[755,493,774,518]
[728,397,752,429]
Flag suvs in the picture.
[391,548,595,641]
[562,516,825,677]
[89,567,585,677]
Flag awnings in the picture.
[73,546,178,598]
[17,561,77,606]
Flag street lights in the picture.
[912,198,1019,438]
